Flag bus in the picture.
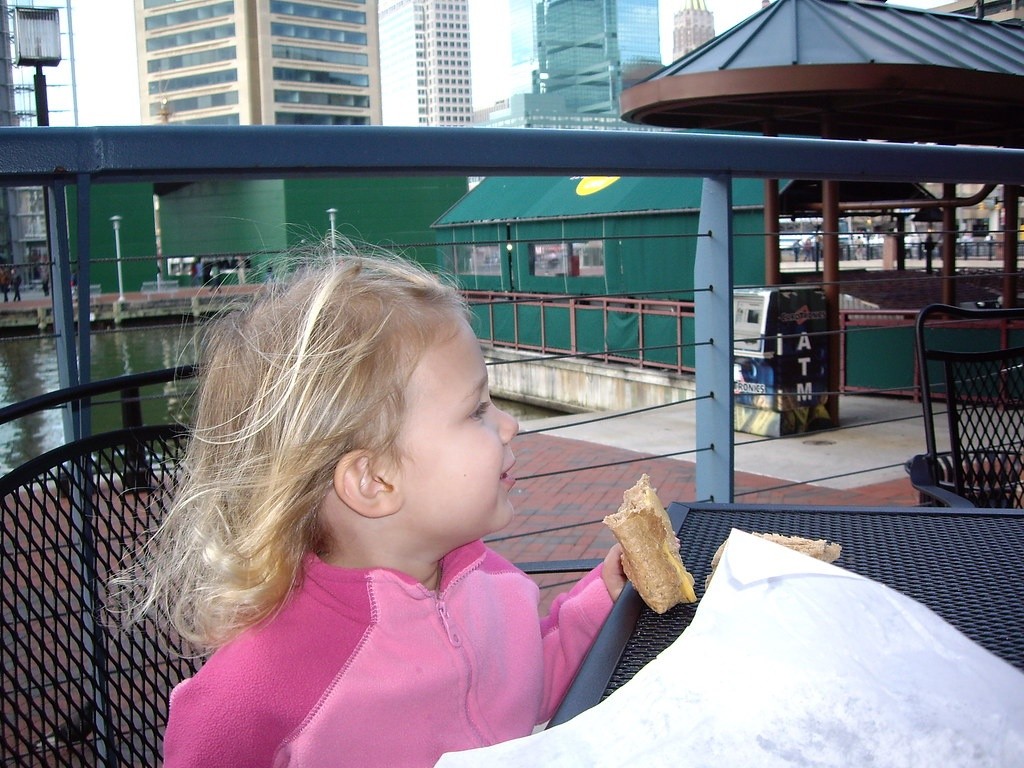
[777,218,853,251]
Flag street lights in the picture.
[108,215,126,302]
[325,207,339,269]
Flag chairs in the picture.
[0,422,214,768]
[903,304,1024,511]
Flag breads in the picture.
[602,474,697,615]
[705,532,842,592]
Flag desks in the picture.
[547,501,1024,730]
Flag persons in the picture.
[793,232,864,263]
[187,258,277,293]
[160,258,680,768]
[0,267,77,302]
